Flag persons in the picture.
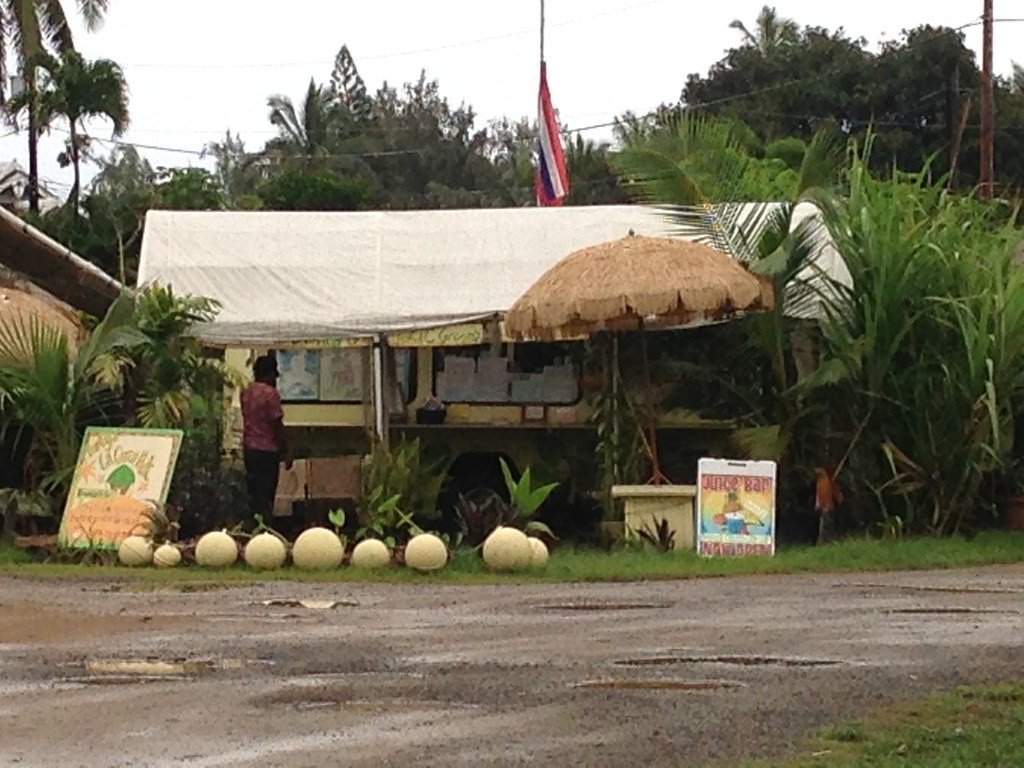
[239,354,295,520]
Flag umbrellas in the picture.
[503,229,775,485]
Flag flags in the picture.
[535,58,574,205]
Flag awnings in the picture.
[134,200,854,339]
